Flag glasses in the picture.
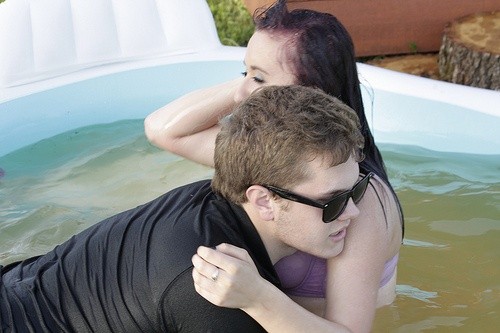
[258,171,372,224]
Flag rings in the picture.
[209,269,221,282]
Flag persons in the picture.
[142,0,405,333]
[0,86,364,333]
